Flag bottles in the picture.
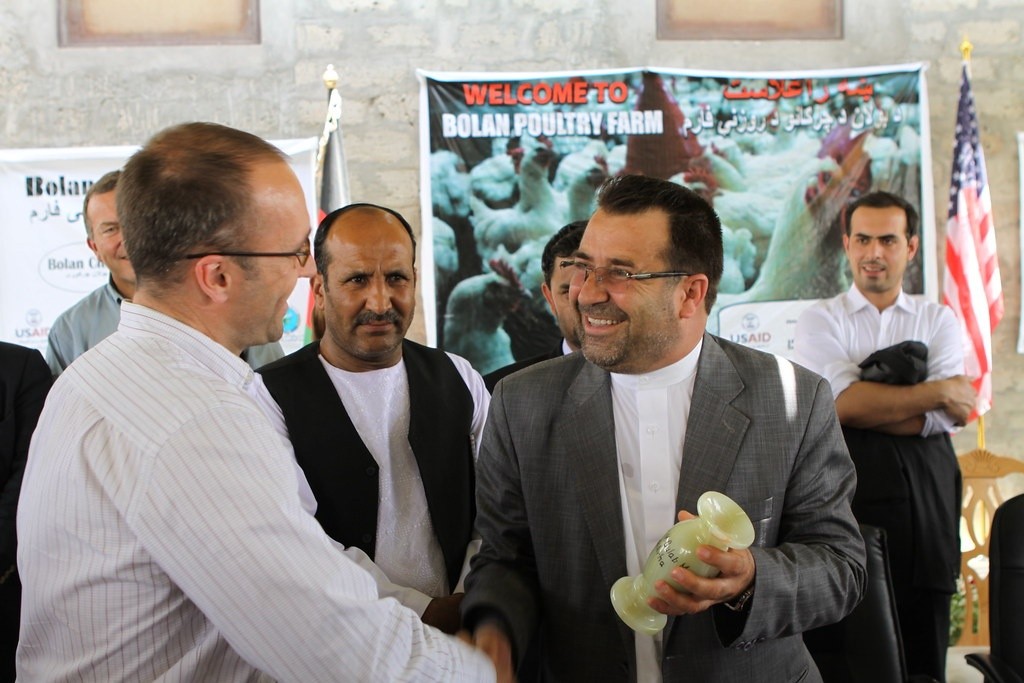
[609,491,756,634]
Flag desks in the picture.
[945,646,991,683]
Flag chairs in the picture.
[955,447,1024,646]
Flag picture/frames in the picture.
[54,0,262,51]
[656,0,845,42]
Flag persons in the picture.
[784,190,983,683]
[255,204,492,635]
[0,122,499,683]
[464,175,870,683]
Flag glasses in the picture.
[559,260,696,293]
[187,238,311,267]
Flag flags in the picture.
[939,64,1007,435]
